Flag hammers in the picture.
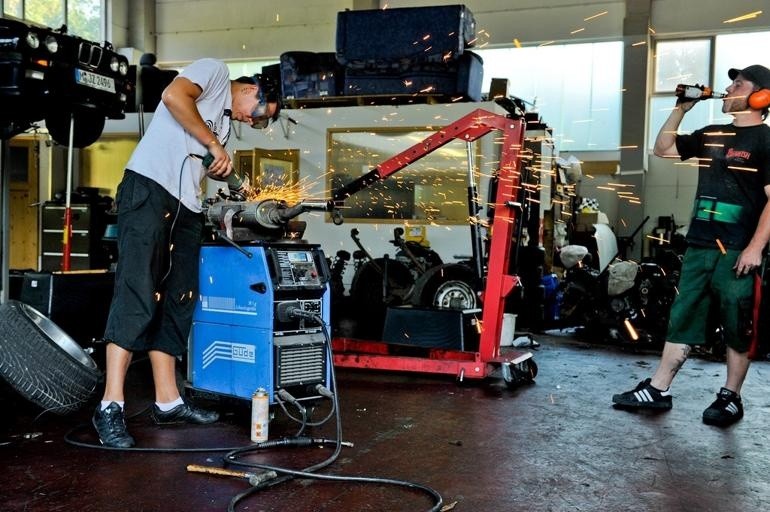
[186,464,278,486]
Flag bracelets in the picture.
[207,139,220,152]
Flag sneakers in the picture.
[699,386,747,427]
[91,402,135,451]
[152,402,221,427]
[611,378,674,410]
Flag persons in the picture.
[613,64,770,431]
[92,57,282,448]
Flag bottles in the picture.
[677,82,728,101]
[249,385,270,445]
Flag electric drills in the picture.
[202,154,256,201]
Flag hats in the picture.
[727,63,770,92]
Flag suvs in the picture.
[0,14,129,148]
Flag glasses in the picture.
[250,100,269,130]
